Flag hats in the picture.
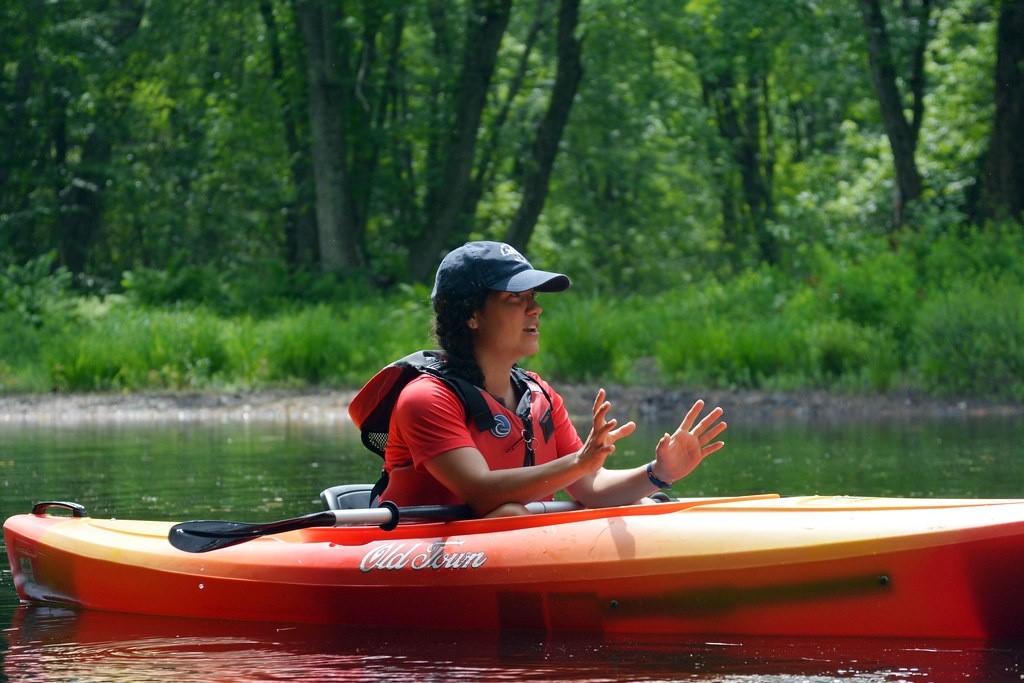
[430,240,571,319]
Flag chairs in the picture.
[319,483,382,509]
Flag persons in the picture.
[375,240,728,519]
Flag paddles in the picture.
[160,491,670,562]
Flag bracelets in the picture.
[646,462,674,491]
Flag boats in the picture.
[2,496,1024,669]
